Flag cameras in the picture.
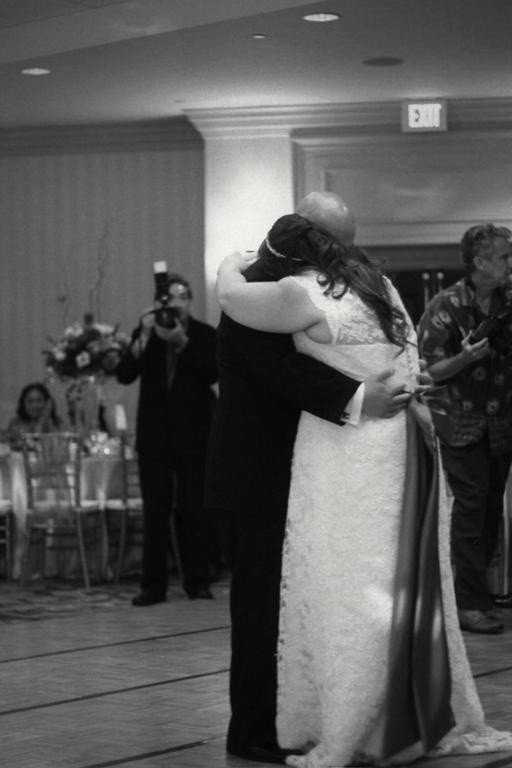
[150,260,179,329]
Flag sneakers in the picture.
[132,583,214,606]
[456,606,506,634]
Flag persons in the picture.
[196,192,436,757]
[215,212,510,767]
[8,382,60,449]
[118,272,216,607]
[416,227,511,634]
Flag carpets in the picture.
[0,561,232,630]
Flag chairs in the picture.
[18,431,144,591]
